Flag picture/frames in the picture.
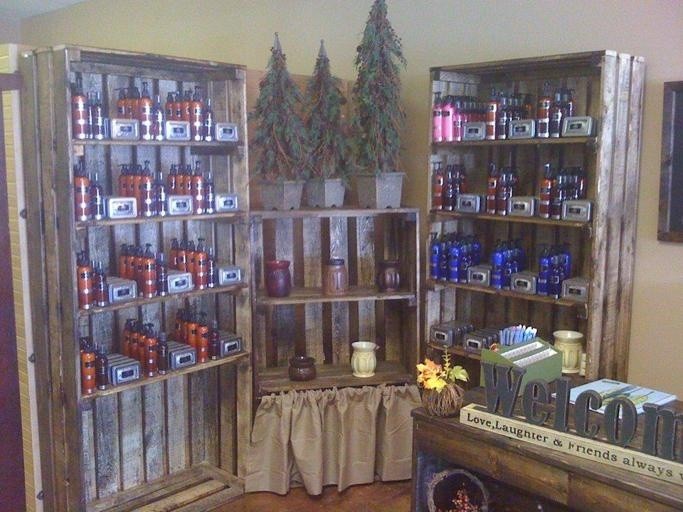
[656,81,683,245]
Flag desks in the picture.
[411,376,683,511]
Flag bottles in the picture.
[430,85,587,298]
[322,259,349,297]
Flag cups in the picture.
[264,260,290,297]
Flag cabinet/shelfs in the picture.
[423,50,648,384]
[19,45,251,512]
[251,206,421,392]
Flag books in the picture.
[551,378,677,420]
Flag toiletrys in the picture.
[80,312,220,394]
[433,158,586,218]
[74,71,218,142]
[432,88,575,144]
[76,159,218,219]
[76,236,214,310]
[431,229,568,299]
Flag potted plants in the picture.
[301,39,352,209]
[353,1,406,209]
[248,32,302,216]
